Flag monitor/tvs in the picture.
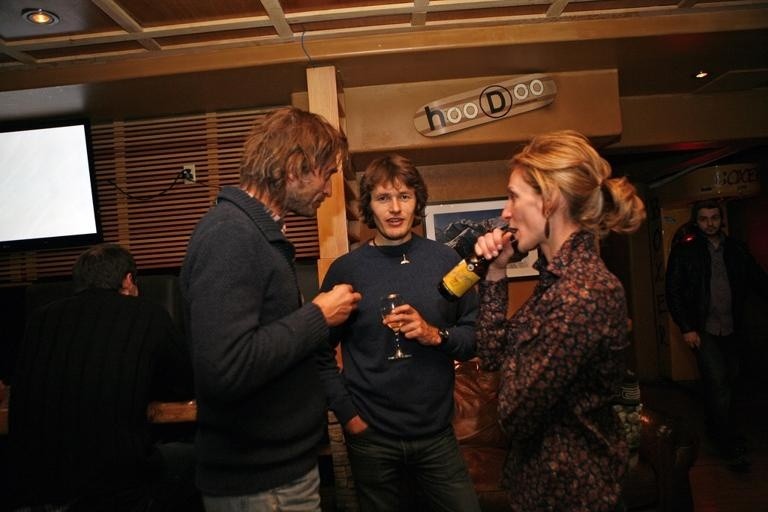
[0,118,104,256]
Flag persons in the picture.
[314,153,484,510]
[170,105,362,511]
[472,128,649,511]
[664,201,767,474]
[2,242,206,512]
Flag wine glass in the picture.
[378,292,414,361]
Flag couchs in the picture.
[452,366,703,511]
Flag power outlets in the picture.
[182,163,197,186]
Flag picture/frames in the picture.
[421,192,545,282]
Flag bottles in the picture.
[437,228,511,304]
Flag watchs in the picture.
[438,327,451,347]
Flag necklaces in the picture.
[372,237,411,265]
